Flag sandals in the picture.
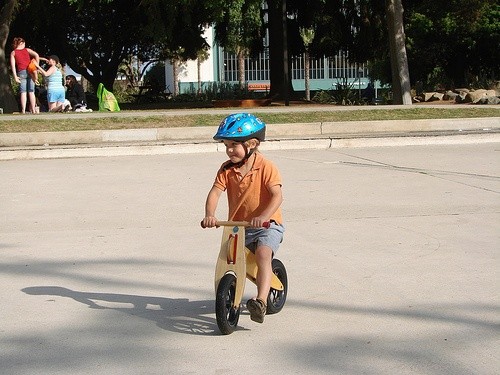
[246,298,268,323]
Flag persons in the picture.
[203,112,284,323]
[10,37,88,115]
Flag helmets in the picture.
[213,113,266,142]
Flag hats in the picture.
[47,55,60,65]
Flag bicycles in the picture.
[201,220,288,336]
